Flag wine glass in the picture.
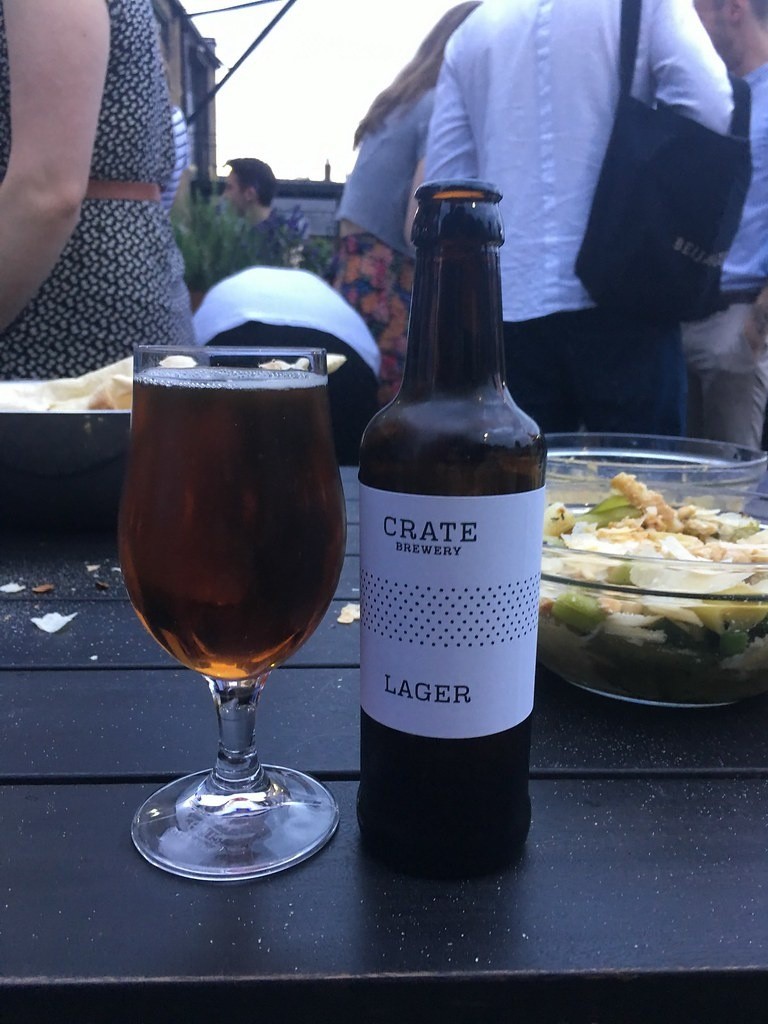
[118,342,342,883]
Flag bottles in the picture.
[357,177,547,878]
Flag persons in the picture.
[191,266,382,465]
[222,155,288,278]
[425,0,735,465]
[336,0,482,410]
[0,0,197,382]
[678,0,768,460]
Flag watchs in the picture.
[748,302,768,326]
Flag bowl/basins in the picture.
[537,430,768,707]
[1,378,132,524]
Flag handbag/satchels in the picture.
[573,0,754,325]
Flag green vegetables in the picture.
[544,494,768,700]
